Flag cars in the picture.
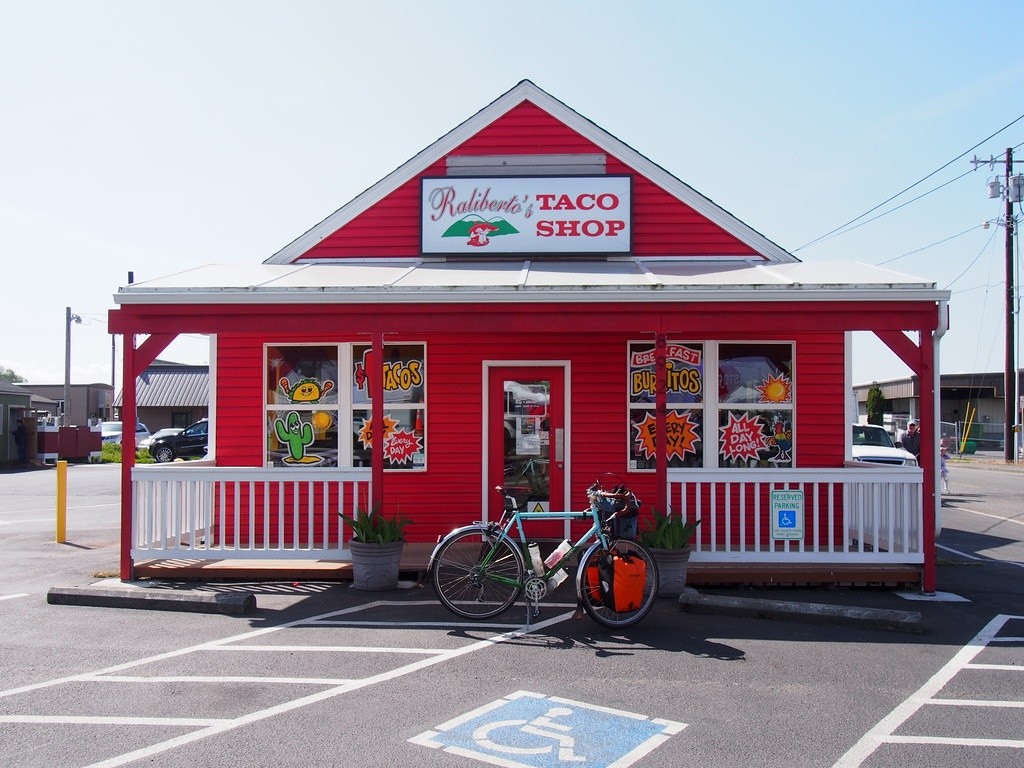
[149,418,209,462]
[96,422,150,448]
[137,427,192,451]
[941,425,962,453]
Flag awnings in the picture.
[111,368,209,407]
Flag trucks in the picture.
[852,391,918,467]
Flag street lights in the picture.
[984,213,1015,463]
[64,314,83,428]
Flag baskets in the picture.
[594,500,639,539]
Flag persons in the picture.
[12,419,26,465]
[899,420,920,467]
[940,433,951,494]
[275,350,337,448]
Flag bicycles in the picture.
[504,448,549,510]
[422,472,660,631]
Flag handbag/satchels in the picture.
[587,548,647,613]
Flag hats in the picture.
[907,420,918,427]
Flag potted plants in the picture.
[337,499,415,591]
[639,505,701,598]
